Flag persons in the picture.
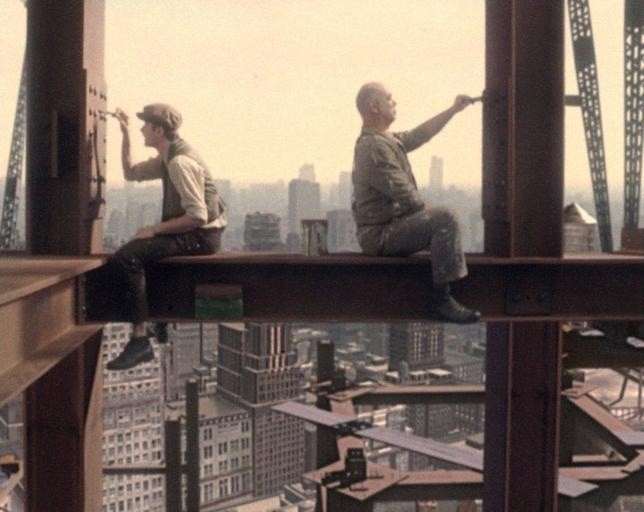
[107,103,227,370]
[352,81,482,323]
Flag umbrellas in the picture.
[422,294,481,324]
[107,323,168,370]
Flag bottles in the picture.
[136,103,182,128]
